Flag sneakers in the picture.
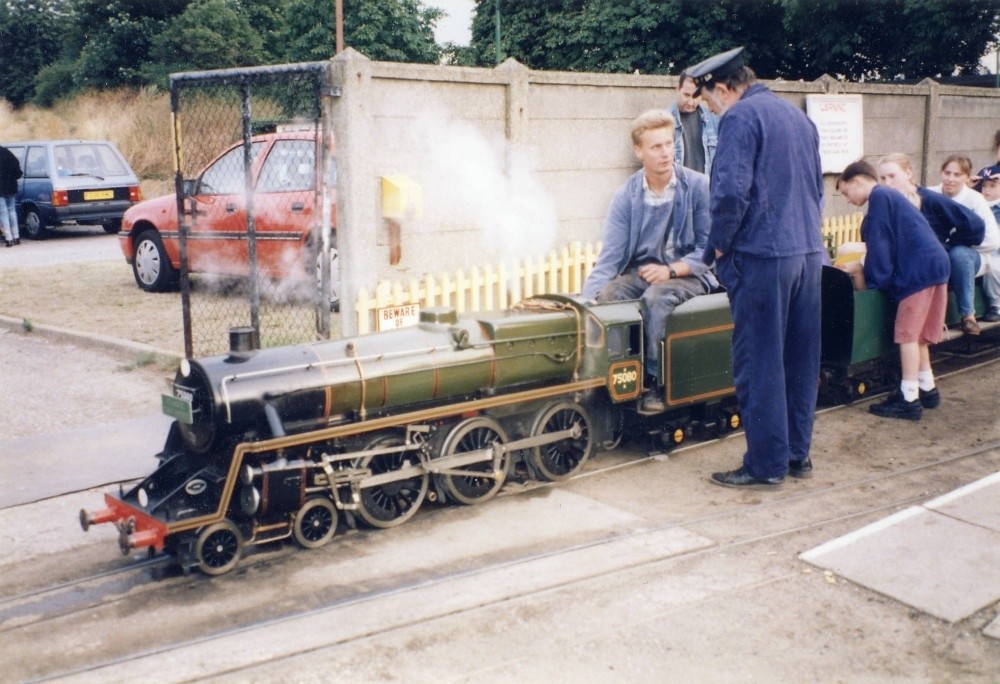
[869,390,922,419]
[887,387,939,409]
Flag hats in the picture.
[972,165,1000,181]
[685,44,745,100]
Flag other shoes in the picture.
[5,239,14,247]
[981,306,1000,323]
[13,238,20,244]
[640,375,664,411]
[961,316,981,336]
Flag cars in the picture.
[0,139,143,242]
[119,129,338,311]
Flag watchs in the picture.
[666,263,676,279]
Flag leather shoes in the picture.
[789,457,813,479]
[711,464,785,490]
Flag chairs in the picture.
[26,156,44,175]
[73,153,95,169]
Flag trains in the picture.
[77,244,1000,582]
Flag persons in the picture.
[580,110,726,411]
[835,161,951,421]
[665,69,721,179]
[0,146,23,247]
[686,48,824,489]
[877,130,1000,336]
